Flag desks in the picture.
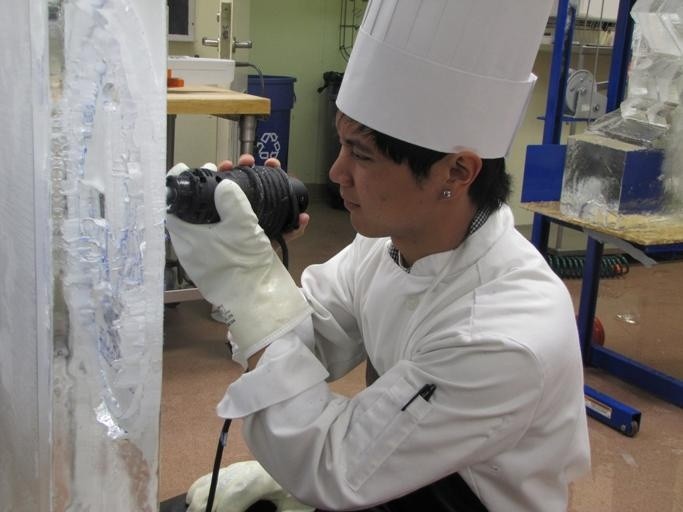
[163,83,271,303]
[514,199,683,436]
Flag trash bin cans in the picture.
[248,75,297,175]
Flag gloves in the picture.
[185,461,315,512]
[166,162,314,363]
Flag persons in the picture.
[167,0,591,512]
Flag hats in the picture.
[334,0,552,160]
